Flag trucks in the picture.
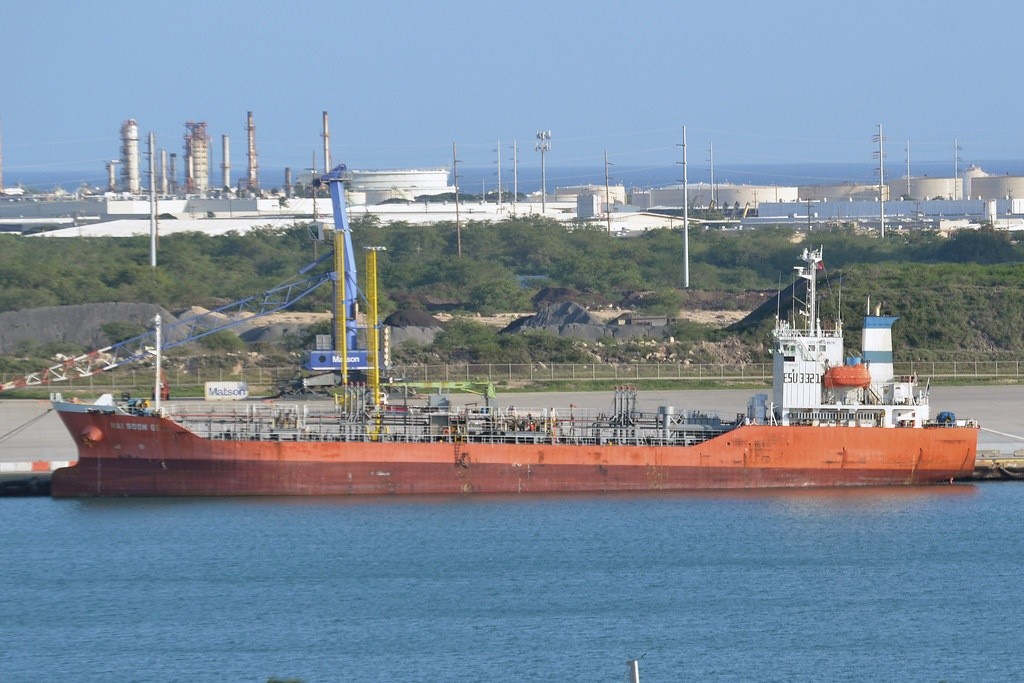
[150,369,170,400]
[335,390,388,412]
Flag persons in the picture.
[509,413,536,431]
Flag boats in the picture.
[48,242,982,513]
